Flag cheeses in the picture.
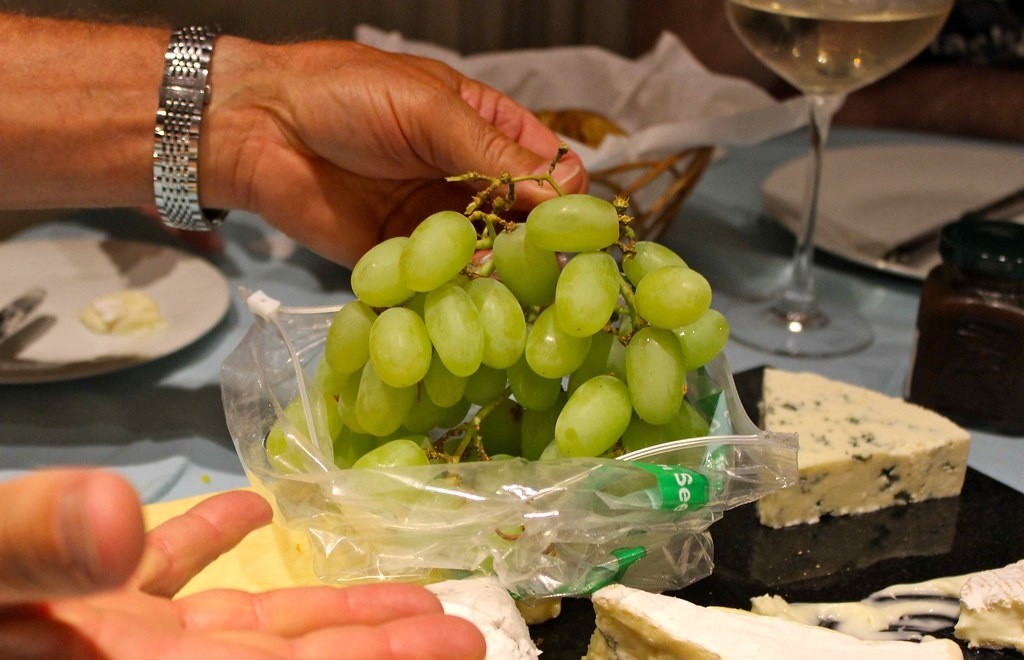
[758,369,972,529]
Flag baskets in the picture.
[581,143,714,242]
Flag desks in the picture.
[0,124,1024,561]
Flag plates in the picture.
[762,146,1024,280]
[0,238,231,383]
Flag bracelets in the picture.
[150,20,228,232]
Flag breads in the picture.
[528,108,628,152]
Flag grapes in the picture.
[266,194,729,554]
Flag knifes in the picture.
[884,188,1024,263]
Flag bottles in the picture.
[903,219,1024,438]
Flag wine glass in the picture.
[724,0,951,358]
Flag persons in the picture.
[633,0,1024,144]
[0,11,588,660]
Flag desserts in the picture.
[77,286,163,335]
[584,559,1024,660]
